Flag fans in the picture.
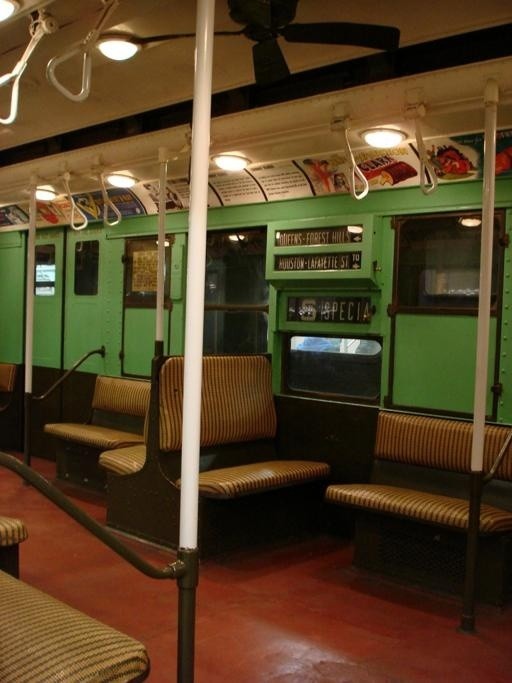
[128,0,401,94]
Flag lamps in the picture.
[95,24,147,62]
[34,184,59,201]
[105,169,138,189]
[460,215,482,228]
[210,153,252,173]
[358,127,408,149]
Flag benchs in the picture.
[0,362,19,448]
[0,567,150,683]
[43,353,329,564]
[0,514,29,579]
[322,412,512,594]
